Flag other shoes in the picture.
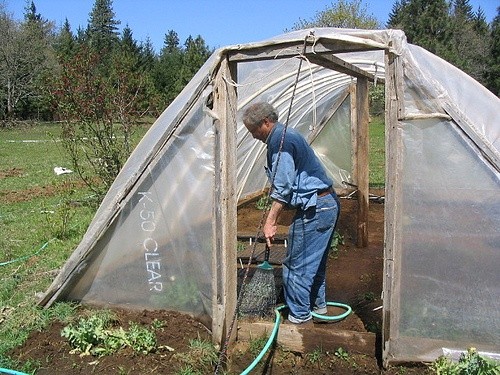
[284,319,315,327]
[313,313,329,324]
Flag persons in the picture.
[242,102,341,328]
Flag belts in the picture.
[317,185,334,197]
